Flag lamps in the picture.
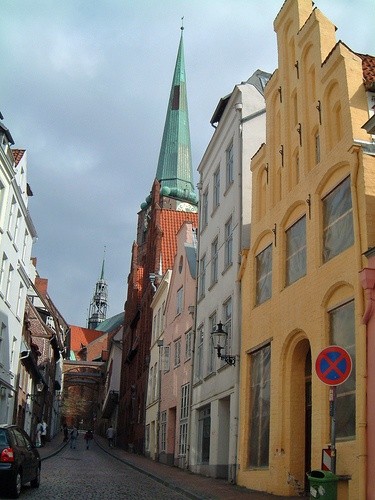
[26,379,44,397]
[211,320,235,366]
[54,392,61,401]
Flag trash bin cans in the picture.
[305,469,352,500]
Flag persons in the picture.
[70,425,79,449]
[106,425,116,449]
[84,429,93,449]
[63,425,69,442]
[35,418,47,448]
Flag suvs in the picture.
[0,424,44,498]
